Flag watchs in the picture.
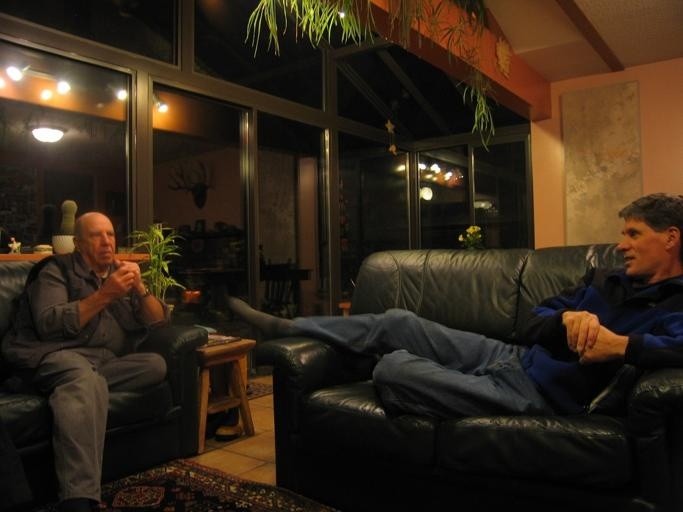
[138,288,152,299]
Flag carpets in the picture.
[36,459,342,512]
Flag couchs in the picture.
[271,245,682,512]
[1,261,208,512]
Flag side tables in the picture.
[197,334,256,454]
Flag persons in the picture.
[0,212,171,511]
[225,192,683,420]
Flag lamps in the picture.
[6,63,169,113]
[29,125,67,143]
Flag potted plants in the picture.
[52,201,78,255]
